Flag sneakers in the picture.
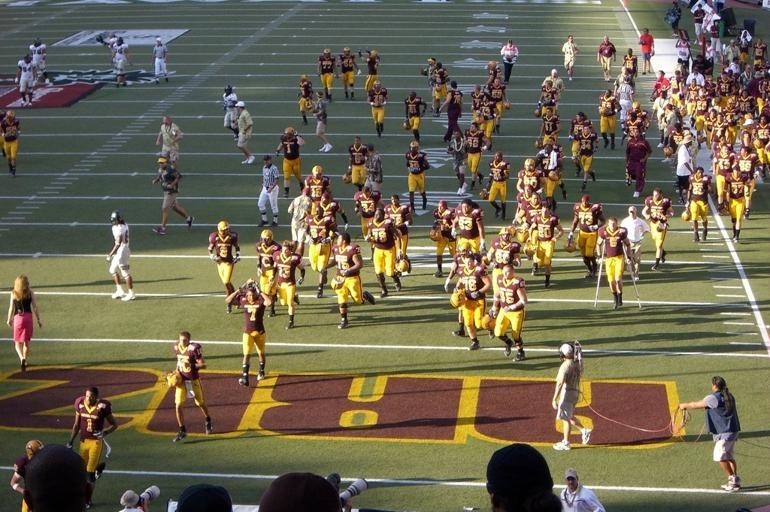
[21,357,28,371]
[95,462,105,479]
[205,420,213,434]
[122,295,136,301]
[582,428,592,444]
[152,226,168,236]
[257,370,265,380]
[553,442,572,451]
[173,431,187,443]
[112,292,124,298]
[213,36,770,360]
[185,215,195,230]
[239,378,250,385]
[721,478,743,491]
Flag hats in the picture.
[564,468,579,479]
[234,101,245,107]
[119,490,140,506]
[157,157,167,163]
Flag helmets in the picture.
[110,210,123,224]
[682,210,691,222]
[25,440,44,459]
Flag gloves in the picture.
[93,432,104,440]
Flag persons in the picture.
[167,331,212,442]
[0,37,47,108]
[6,274,42,372]
[678,375,742,491]
[1,110,21,176]
[104,32,129,87]
[154,36,169,84]
[639,0,769,243]
[9,439,351,512]
[488,442,606,512]
[107,211,136,301]
[209,47,430,387]
[153,117,195,234]
[66,387,118,507]
[550,343,592,450]
[419,35,651,362]
